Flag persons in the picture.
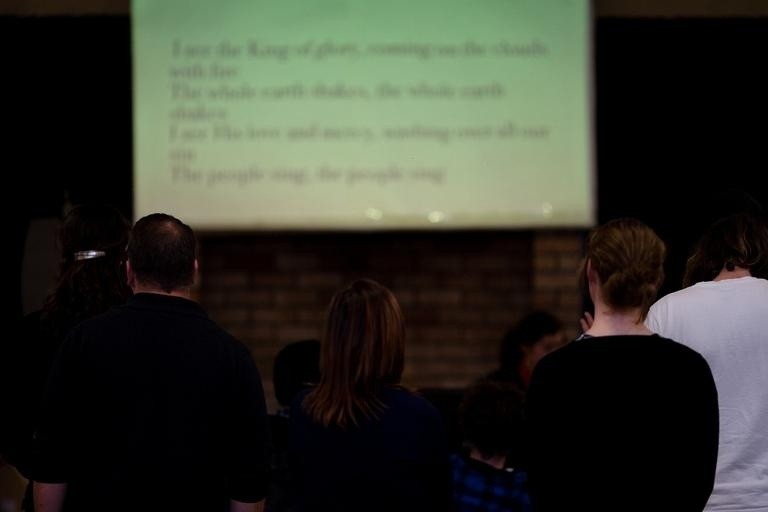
[0,208,268,511]
[266,278,448,511]
[510,214,768,510]
[443,311,564,509]
